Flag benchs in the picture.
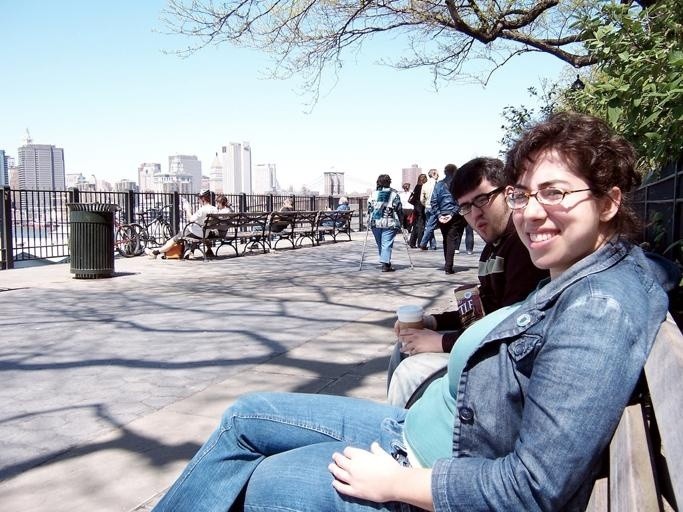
[173,210,354,264]
[565,310,683,512]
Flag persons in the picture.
[144,190,219,259]
[315,198,351,241]
[385,158,550,400]
[182,194,232,260]
[246,197,294,250]
[146,106,670,512]
[365,163,473,274]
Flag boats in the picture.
[27,220,59,230]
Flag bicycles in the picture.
[114,202,175,258]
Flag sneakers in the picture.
[444,267,454,274]
[454,249,473,255]
[143,247,157,260]
[381,265,395,272]
[401,241,437,252]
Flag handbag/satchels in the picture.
[407,210,416,225]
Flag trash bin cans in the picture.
[65,203,122,278]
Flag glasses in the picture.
[505,185,594,210]
[457,186,506,217]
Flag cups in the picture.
[397,304,424,355]
